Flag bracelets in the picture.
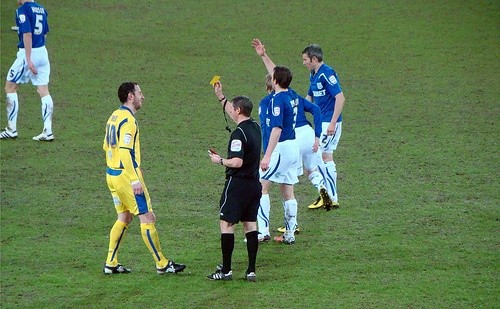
[219,96,225,102]
[131,180,139,185]
[219,158,223,165]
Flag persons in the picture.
[205,81,262,282]
[102,82,187,275]
[0,0,54,142]
[244,38,300,245]
[259,72,332,233]
[302,44,345,211]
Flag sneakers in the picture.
[277,226,300,234]
[0,127,18,139]
[246,271,256,282]
[206,264,232,281]
[244,233,270,242]
[156,261,186,275]
[307,194,323,209]
[319,185,333,211]
[274,235,295,244]
[332,201,340,208]
[32,132,54,141]
[104,263,132,275]
